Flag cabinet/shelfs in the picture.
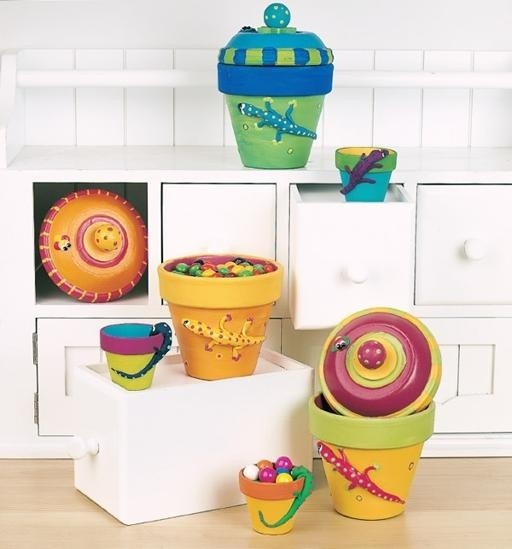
[0,144,512,460]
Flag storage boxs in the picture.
[288,183,417,332]
[71,345,316,526]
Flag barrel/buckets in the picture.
[334,147,397,203]
[218,63,334,170]
[238,462,314,536]
[307,389,436,520]
[99,323,172,392]
[157,251,284,382]
